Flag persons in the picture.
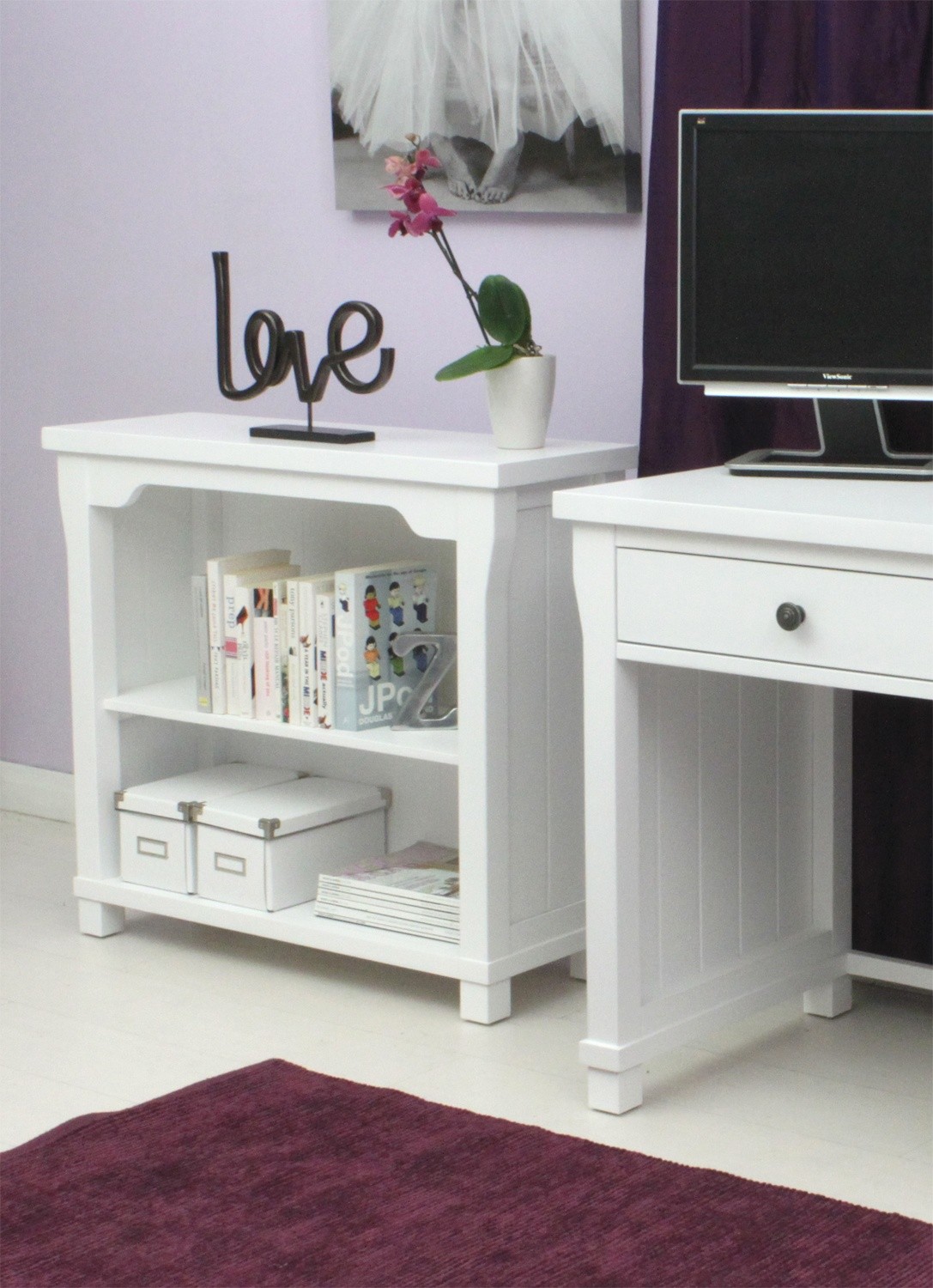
[332,1,641,205]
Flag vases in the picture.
[485,355,556,450]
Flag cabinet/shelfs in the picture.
[41,412,640,1025]
[553,465,933,1115]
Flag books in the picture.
[317,839,460,944]
[190,548,438,734]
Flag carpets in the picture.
[0,1058,933,1287]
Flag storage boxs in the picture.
[114,762,308,894]
[191,776,392,912]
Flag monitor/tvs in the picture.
[677,110,933,484]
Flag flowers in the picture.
[380,133,542,381]
